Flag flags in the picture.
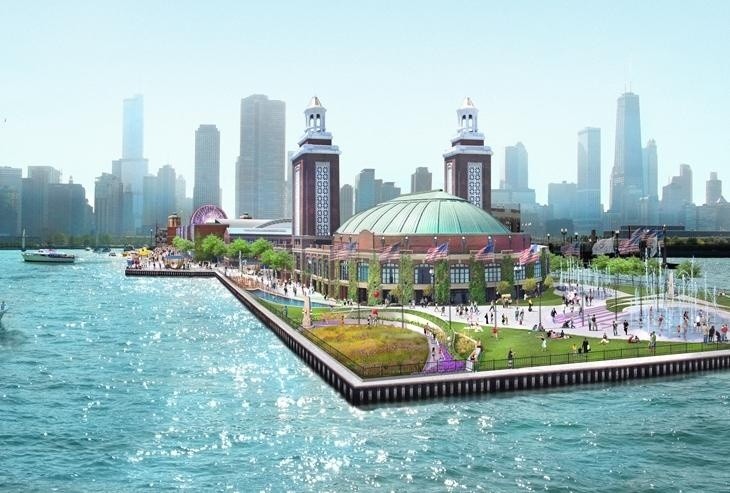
[475,243,494,262]
[591,236,615,255]
[519,244,540,265]
[429,243,448,261]
[336,242,358,257]
[617,227,665,256]
[379,241,401,266]
[560,241,580,258]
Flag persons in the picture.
[138,243,218,270]
[255,270,353,305]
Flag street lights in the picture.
[547,233,551,245]
[536,281,542,331]
[151,229,153,246]
[560,228,567,247]
[615,230,620,258]
[575,232,578,240]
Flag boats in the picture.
[85,246,116,256]
[21,248,76,263]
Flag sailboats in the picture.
[21,229,26,251]
